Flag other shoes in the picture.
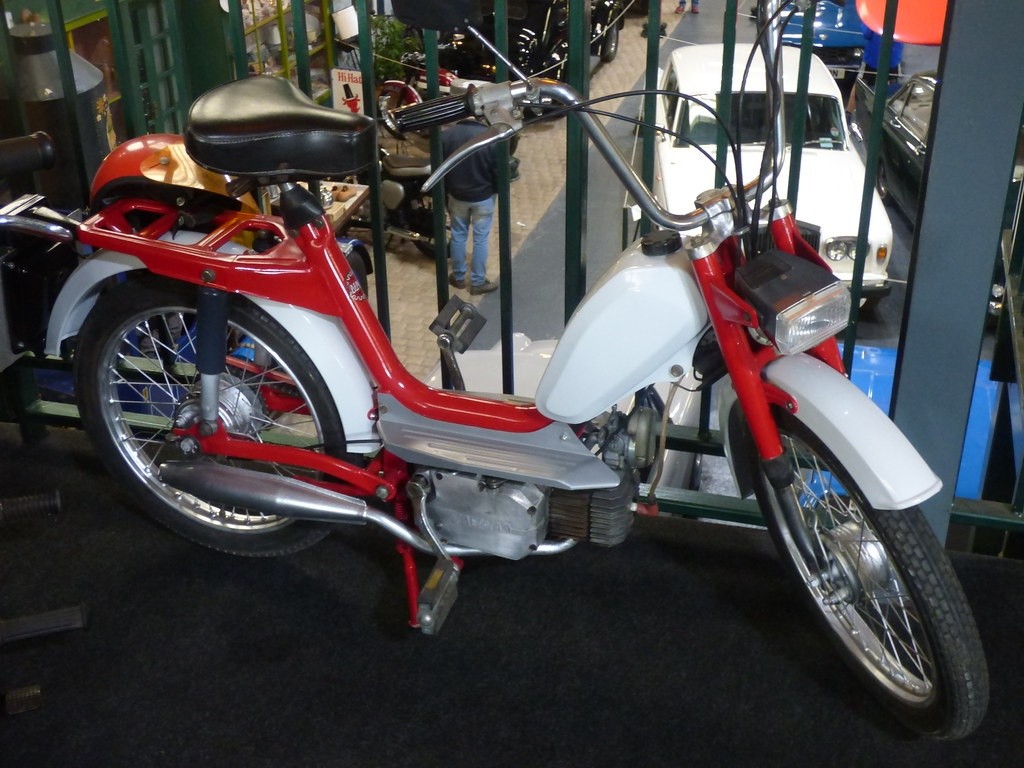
[448,273,465,289]
[675,7,684,14]
[471,284,499,295]
[692,6,700,14]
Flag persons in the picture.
[861,22,905,87]
[673,0,700,15]
[438,113,499,296]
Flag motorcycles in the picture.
[1,72,992,747]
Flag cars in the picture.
[653,43,894,319]
[847,70,1024,331]
[451,0,627,82]
